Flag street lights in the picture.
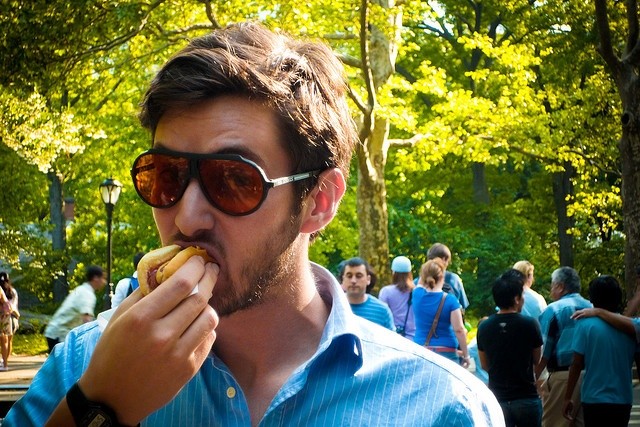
[99,174,121,309]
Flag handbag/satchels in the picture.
[396,325,405,337]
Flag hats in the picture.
[390,256,413,274]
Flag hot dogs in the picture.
[137,243,215,296]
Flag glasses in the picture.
[128,146,321,219]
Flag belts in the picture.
[426,345,457,353]
[552,366,569,372]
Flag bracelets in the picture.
[463,355,470,361]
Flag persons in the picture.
[477,269,543,426]
[561,275,639,427]
[495,260,548,425]
[111,253,143,308]
[534,267,594,427]
[341,259,396,333]
[0,272,19,370]
[380,257,415,339]
[337,261,346,293]
[412,259,471,369]
[44,266,108,354]
[0,286,21,320]
[570,307,640,332]
[413,242,471,362]
[0,23,507,427]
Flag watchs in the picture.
[67,382,142,427]
